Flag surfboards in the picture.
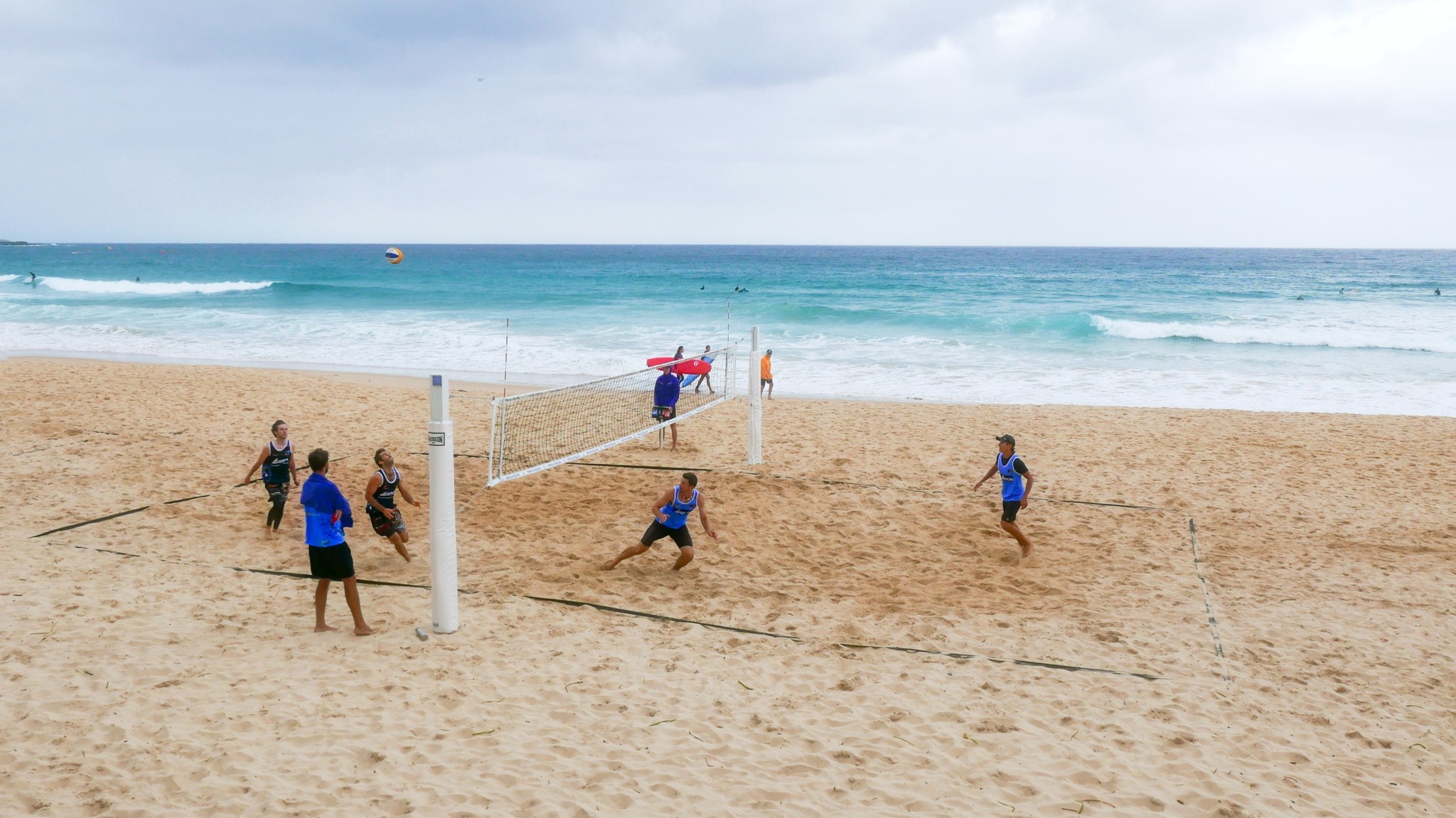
[647,357,712,375]
[681,351,718,389]
[21,277,45,284]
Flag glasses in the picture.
[768,352,772,354]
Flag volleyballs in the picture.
[385,247,403,264]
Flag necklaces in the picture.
[683,490,693,501]
[385,470,394,475]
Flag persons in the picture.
[365,448,422,563]
[301,448,378,635]
[605,472,718,571]
[674,346,684,383]
[695,345,715,394]
[244,420,300,540]
[973,434,1034,558]
[654,365,680,454]
[760,349,775,400]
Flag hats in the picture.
[996,434,1015,446]
